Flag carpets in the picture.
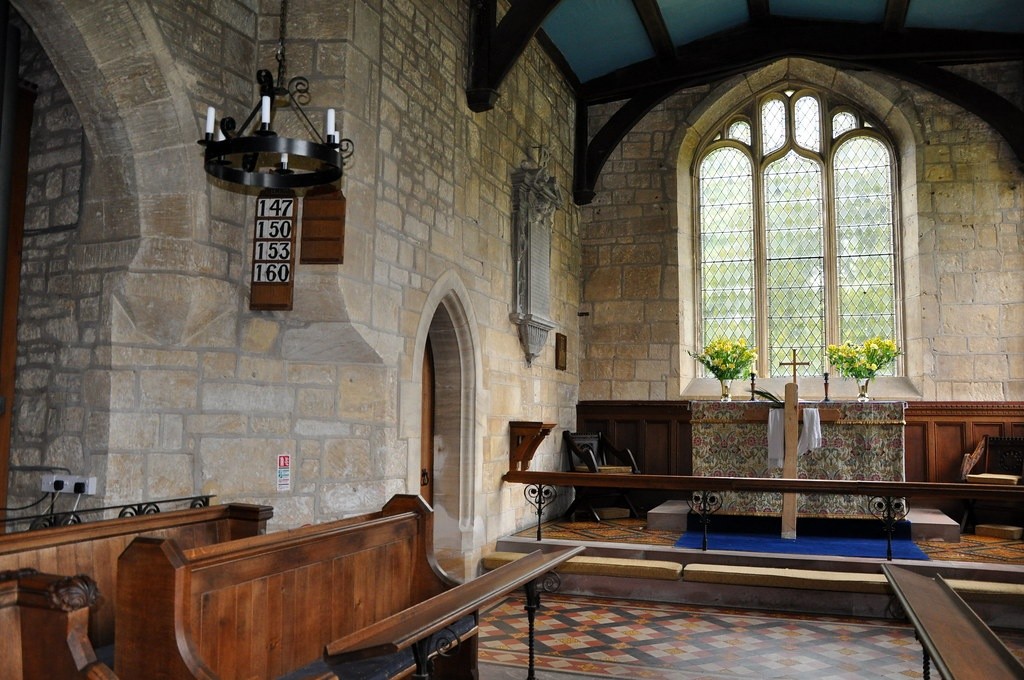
[675,529,931,561]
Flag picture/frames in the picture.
[556,333,567,371]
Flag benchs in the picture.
[0,502,585,680]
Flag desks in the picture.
[689,400,908,519]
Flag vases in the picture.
[721,378,732,402]
[857,377,869,402]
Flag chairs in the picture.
[960,434,1024,533]
[561,430,641,522]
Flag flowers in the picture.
[826,336,900,376]
[685,334,759,381]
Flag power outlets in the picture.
[39,474,97,496]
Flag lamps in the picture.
[197,0,355,188]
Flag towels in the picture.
[767,408,785,469]
[797,407,822,455]
